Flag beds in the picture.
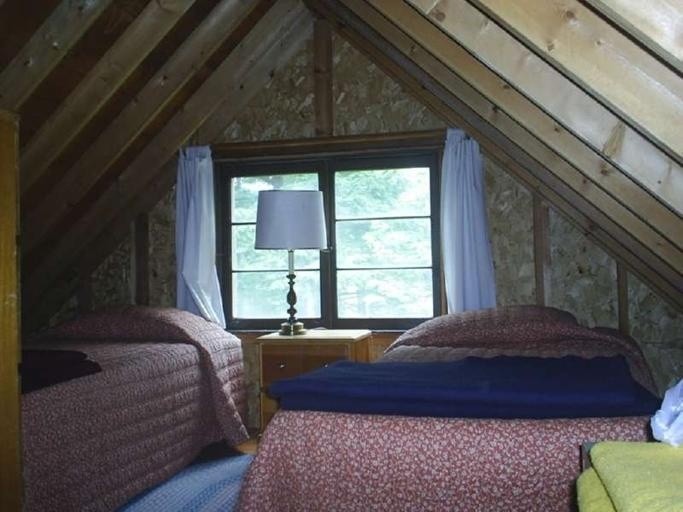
[19,307,247,512]
[238,305,659,510]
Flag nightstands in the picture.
[257,330,374,430]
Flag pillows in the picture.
[53,304,204,342]
[408,305,578,348]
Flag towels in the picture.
[574,439,683,512]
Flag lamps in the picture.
[255,190,329,334]
